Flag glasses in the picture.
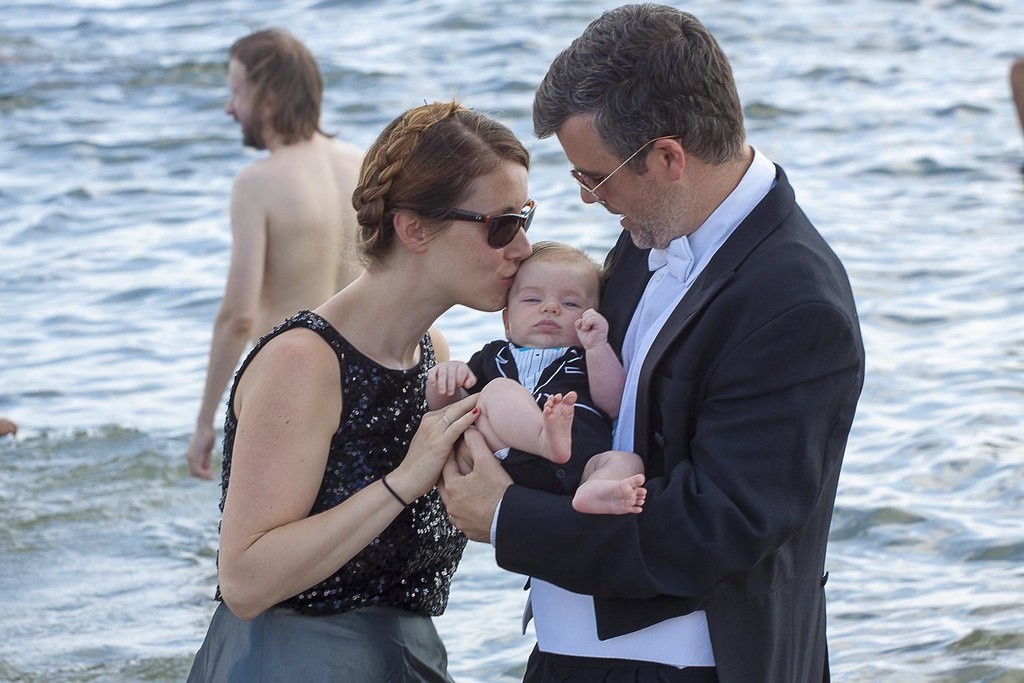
[446,197,537,250]
[569,132,683,203]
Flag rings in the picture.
[448,514,452,523]
[442,415,450,424]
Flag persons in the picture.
[0,418,17,436]
[183,3,865,683]
[1011,60,1024,129]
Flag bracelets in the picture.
[381,475,408,508]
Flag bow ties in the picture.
[648,236,695,283]
